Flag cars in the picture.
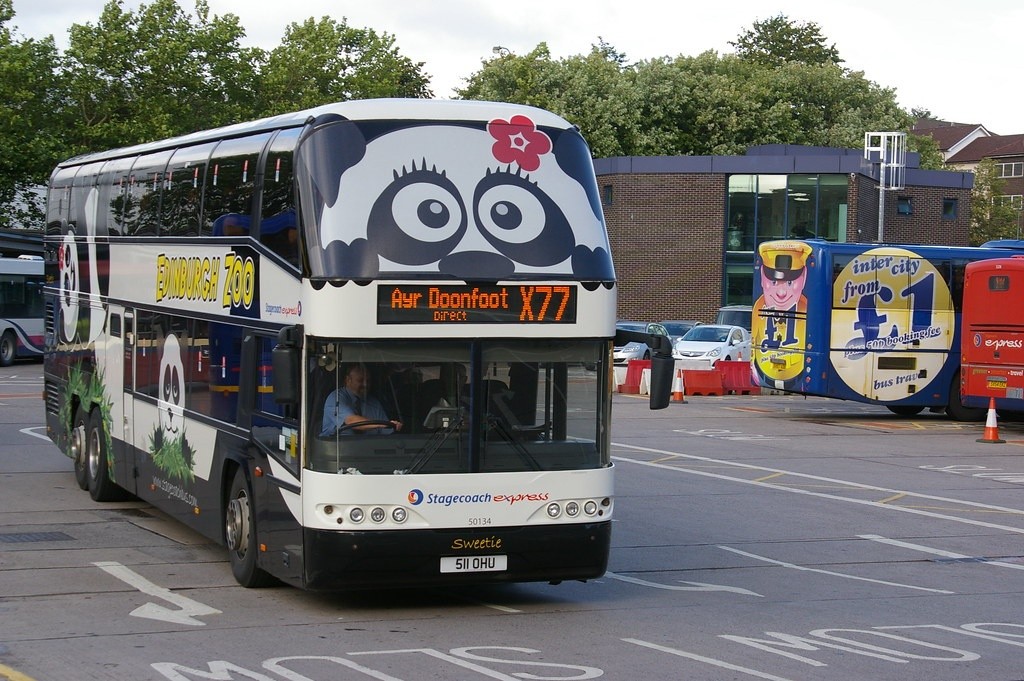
[657,320,704,345]
[716,307,754,338]
[608,318,673,366]
[671,325,750,365]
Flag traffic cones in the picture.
[975,397,1006,444]
[669,369,688,404]
[736,352,743,361]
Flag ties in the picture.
[353,397,363,435]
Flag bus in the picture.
[752,236,1024,421]
[0,255,45,366]
[43,96,675,605]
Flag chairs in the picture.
[140,192,296,251]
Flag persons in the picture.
[323,359,500,444]
